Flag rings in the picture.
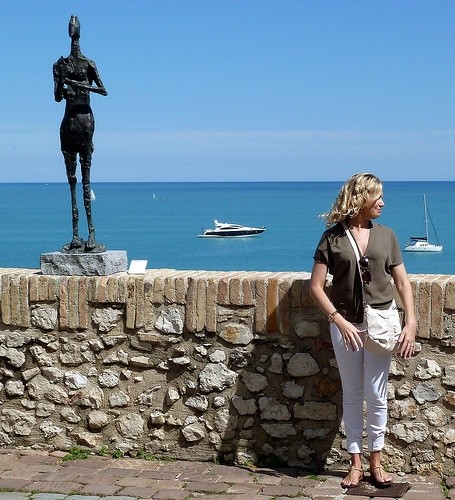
[410,344,414,347]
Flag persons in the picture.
[51,14,110,254]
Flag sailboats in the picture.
[401,194,443,252]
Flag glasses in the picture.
[359,256,371,285]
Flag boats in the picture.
[197,219,265,238]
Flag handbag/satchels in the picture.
[363,298,401,352]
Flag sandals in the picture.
[341,466,364,487]
[370,464,393,485]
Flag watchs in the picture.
[308,173,419,489]
[328,310,339,324]
